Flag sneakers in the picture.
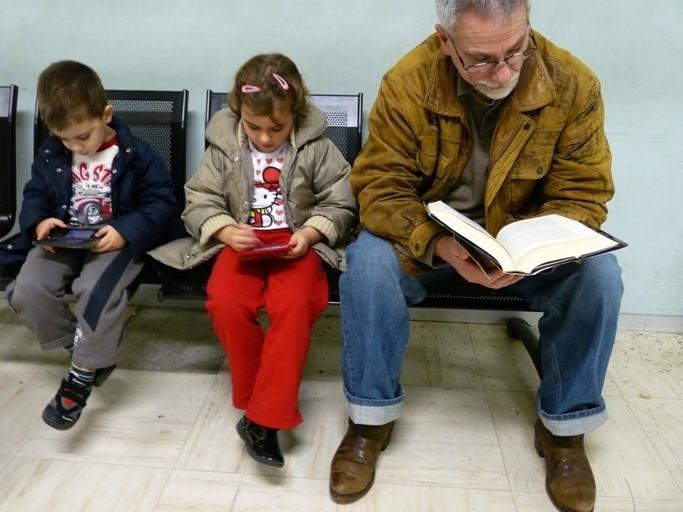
[42,346,116,430]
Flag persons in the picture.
[179,52,359,468]
[4,58,180,432]
[327,0,625,511]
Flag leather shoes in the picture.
[236,416,285,467]
[330,417,394,504]
[534,418,595,512]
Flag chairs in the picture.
[0,83,545,382]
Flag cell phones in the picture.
[244,243,290,259]
[31,224,103,250]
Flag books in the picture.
[421,198,627,278]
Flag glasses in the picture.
[448,20,539,73]
